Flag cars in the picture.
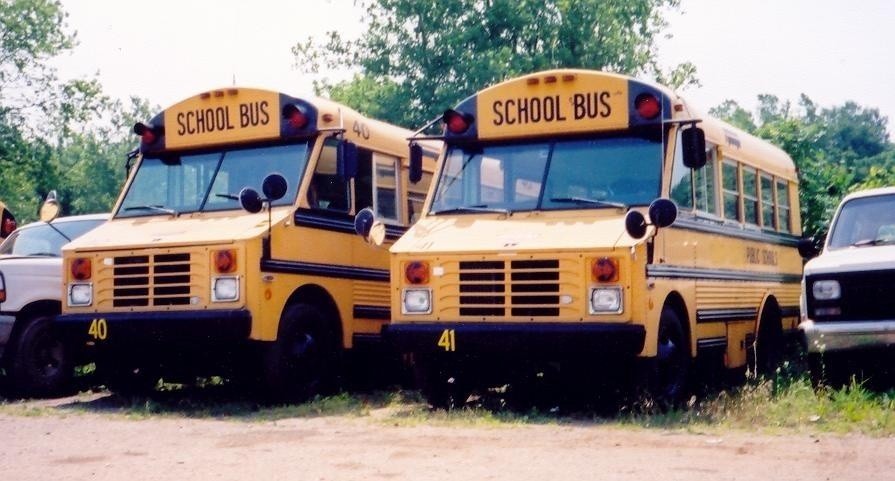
[0,213,112,399]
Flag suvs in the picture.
[795,187,890,384]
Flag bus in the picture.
[65,87,444,405]
[376,66,806,411]
